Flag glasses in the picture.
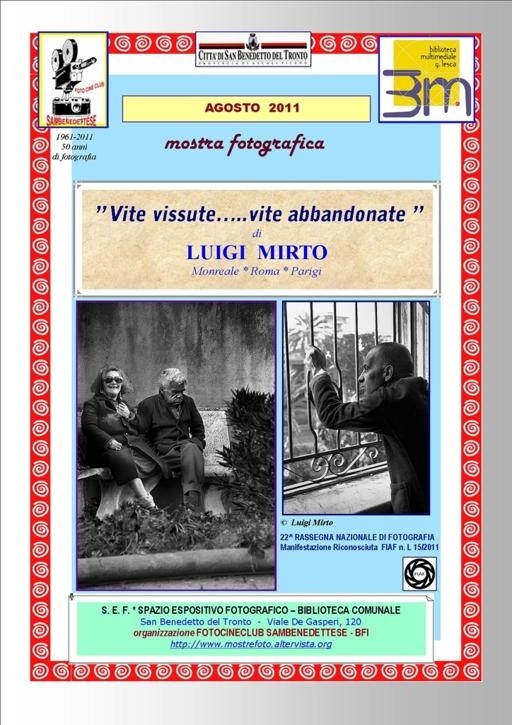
[102,377,122,383]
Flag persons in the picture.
[304,342,430,515]
[81,364,159,512]
[136,367,206,511]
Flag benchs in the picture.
[78,411,232,523]
